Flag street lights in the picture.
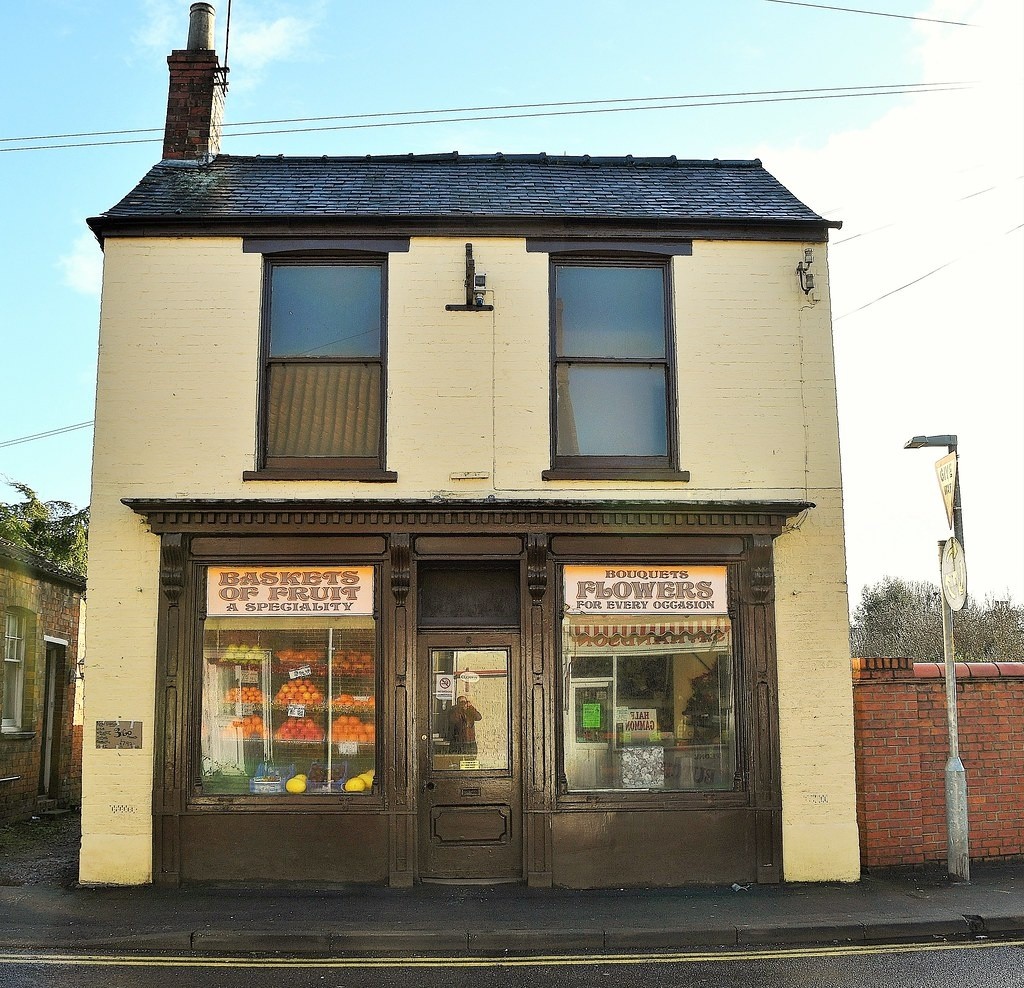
[902,431,972,888]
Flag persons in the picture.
[448,696,483,755]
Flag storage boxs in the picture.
[250,760,296,795]
[304,756,348,794]
